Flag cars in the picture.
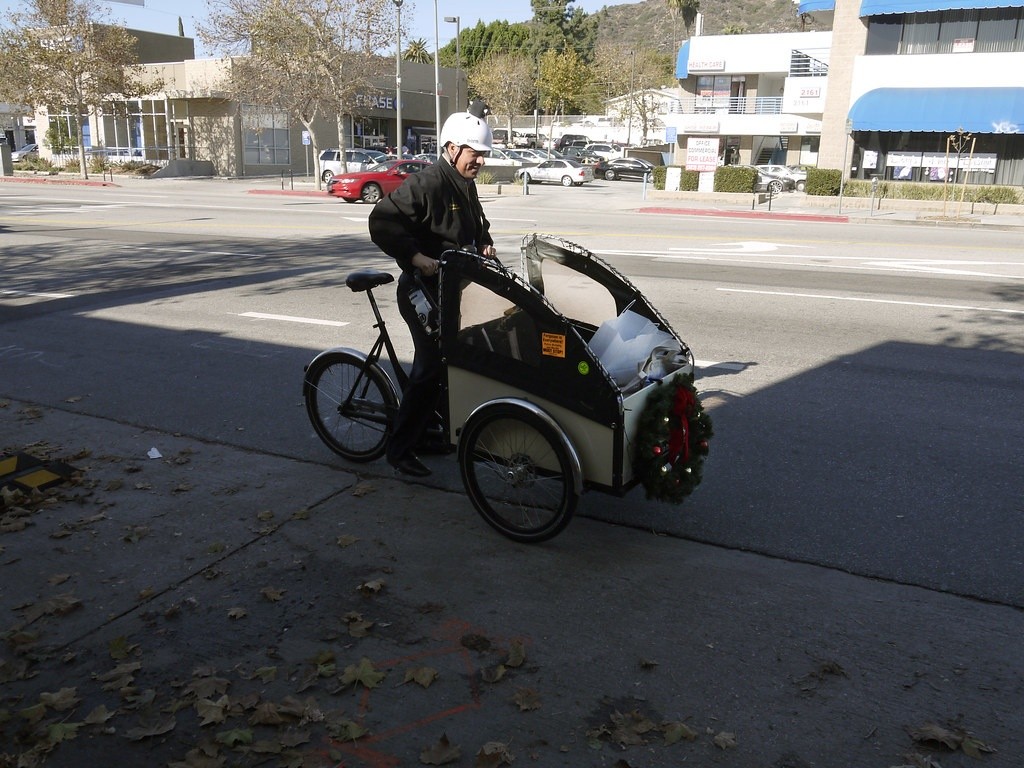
[727,163,813,194]
[483,128,624,166]
[327,159,434,204]
[10,144,39,162]
[374,153,437,169]
[595,157,656,184]
[515,159,593,187]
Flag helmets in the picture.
[440,113,493,151]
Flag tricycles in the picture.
[302,231,713,546]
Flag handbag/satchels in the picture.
[587,300,673,386]
[620,340,688,394]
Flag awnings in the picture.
[797,0,836,15]
[674,40,689,82]
[859,0,1024,19]
[847,87,1024,136]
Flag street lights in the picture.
[392,0,404,159]
[444,16,459,113]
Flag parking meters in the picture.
[870,176,878,216]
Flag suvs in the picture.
[319,148,394,183]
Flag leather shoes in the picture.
[387,452,433,477]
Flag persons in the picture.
[367,111,497,477]
